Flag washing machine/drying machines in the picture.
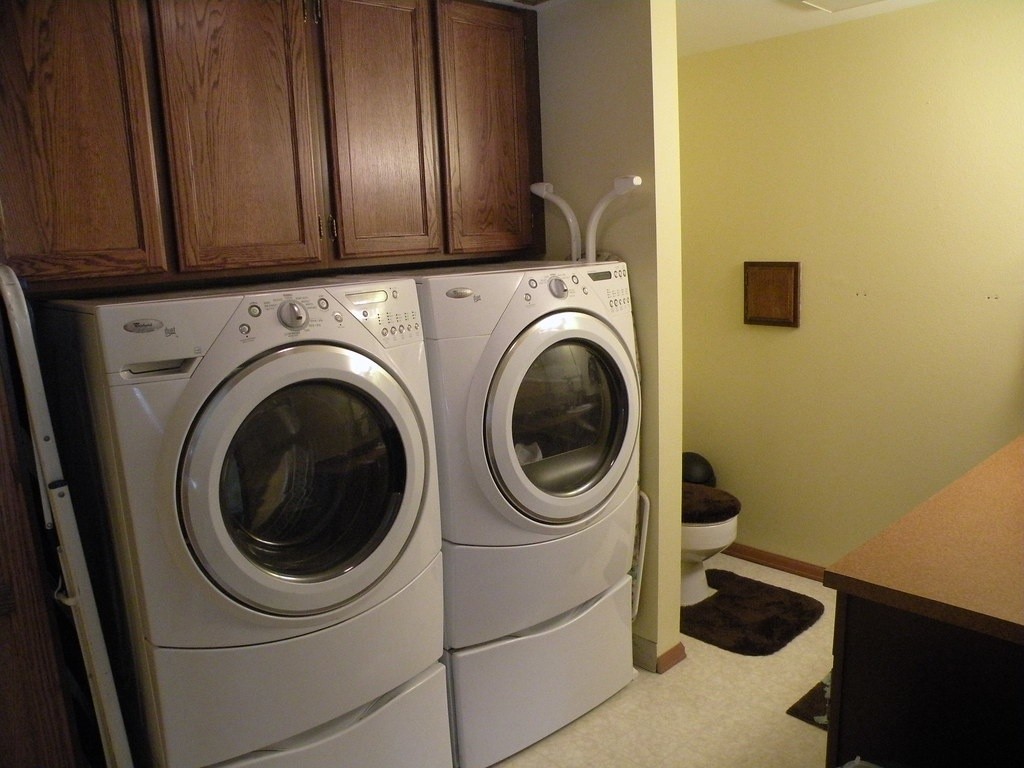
[2,253,644,768]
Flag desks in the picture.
[821,430,1024,767]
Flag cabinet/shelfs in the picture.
[1,0,326,298]
[321,0,547,270]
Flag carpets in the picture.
[680,567,825,658]
[785,665,833,731]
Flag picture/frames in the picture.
[743,261,800,329]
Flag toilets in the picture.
[681,479,741,609]
